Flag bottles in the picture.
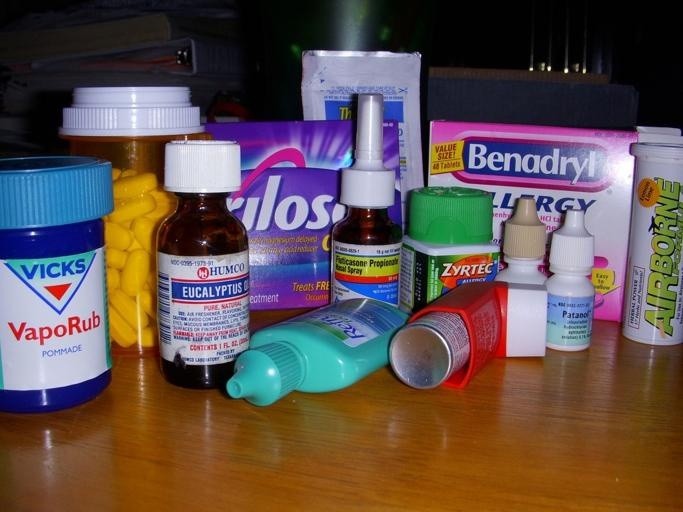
[387,316,478,391]
[546,206,595,352]
[0,152,115,416]
[223,299,416,403]
[495,195,546,358]
[61,84,210,352]
[328,85,401,305]
[153,138,251,388]
[399,184,498,315]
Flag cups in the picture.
[621,139,682,347]
